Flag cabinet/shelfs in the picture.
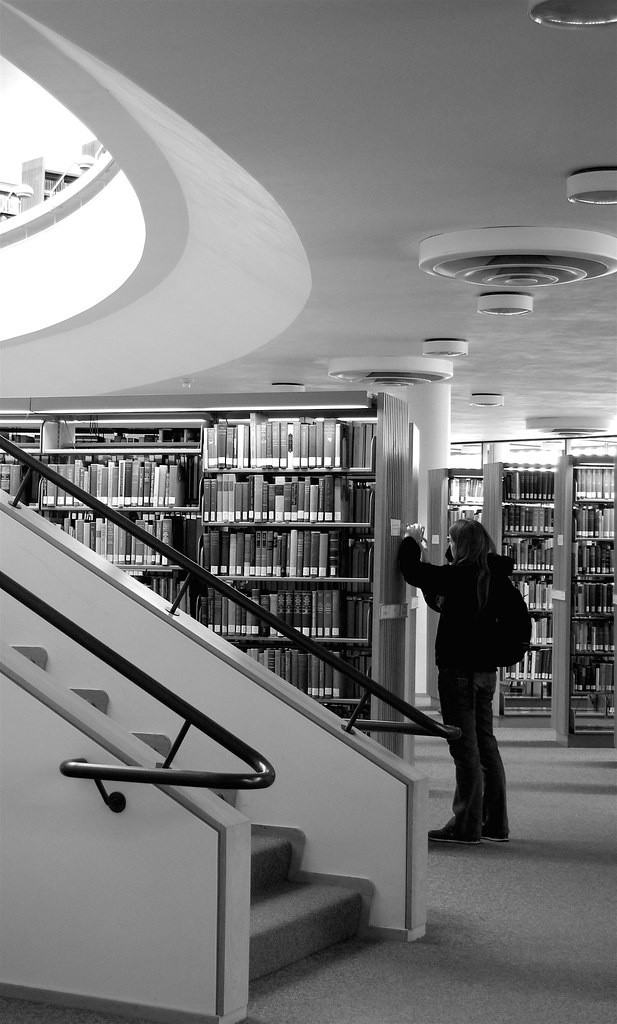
[426,468,484,710]
[20,158,80,215]
[0,410,421,760]
[554,455,617,748]
[482,463,613,716]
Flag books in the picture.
[2,412,615,737]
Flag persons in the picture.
[394,520,509,843]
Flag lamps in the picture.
[568,167,617,206]
[423,338,469,358]
[49,153,94,195]
[468,393,505,409]
[6,182,35,209]
[476,292,533,318]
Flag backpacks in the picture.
[473,569,532,668]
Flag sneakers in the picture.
[480,827,510,842]
[428,825,482,844]
